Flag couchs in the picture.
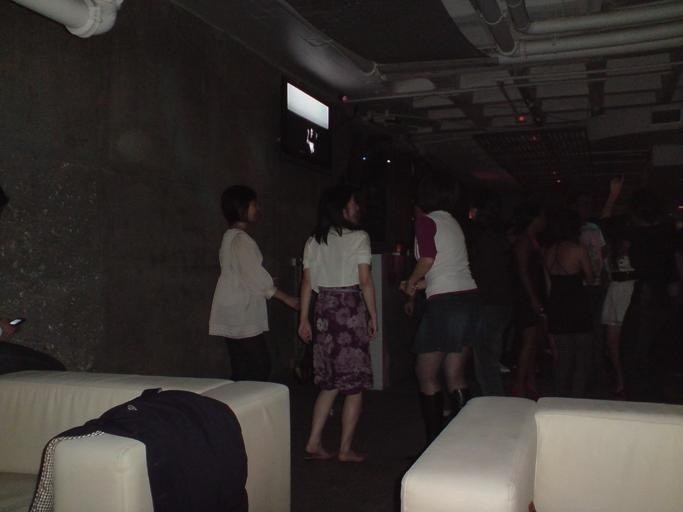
[0,370,291,512]
[399,395,683,512]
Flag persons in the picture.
[0,187,66,376]
[402,172,682,448]
[209,185,301,382]
[298,188,378,463]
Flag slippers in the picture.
[338,450,368,464]
[303,442,335,461]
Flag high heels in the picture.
[521,379,542,402]
[502,379,525,396]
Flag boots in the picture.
[421,388,446,443]
[449,386,469,417]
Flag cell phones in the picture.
[8,316,27,327]
